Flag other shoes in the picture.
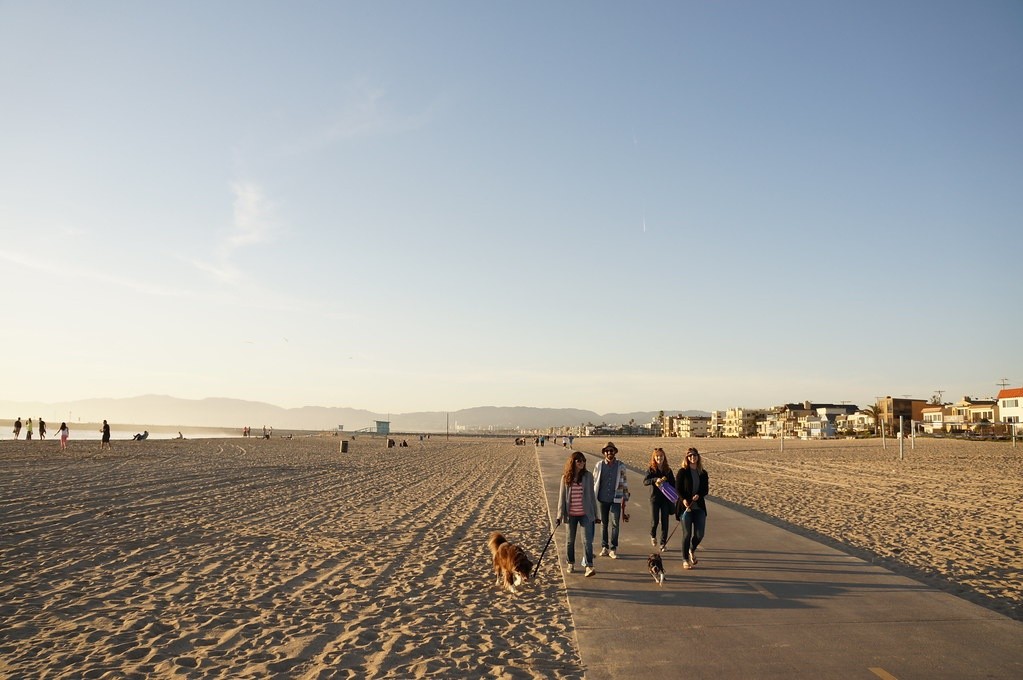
[567,564,574,573]
[682,561,692,569]
[599,548,608,556]
[689,549,697,565]
[584,568,595,577]
[650,536,657,546]
[658,545,666,552]
[608,551,619,559]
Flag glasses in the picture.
[654,448,662,451]
[575,459,586,462]
[603,449,616,453]
[687,453,698,457]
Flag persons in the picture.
[179,431,183,439]
[132,431,147,440]
[100,420,111,450]
[593,442,629,558]
[14,417,46,440]
[558,451,598,577]
[54,423,69,449]
[534,433,558,446]
[262,425,272,438]
[644,449,675,552]
[675,448,709,569]
[563,433,574,450]
[244,426,251,436]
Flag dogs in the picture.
[648,553,665,587]
[488,531,532,593]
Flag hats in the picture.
[601,442,618,454]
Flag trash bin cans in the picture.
[387,439,393,448]
[420,435,423,440]
[339,441,348,453]
